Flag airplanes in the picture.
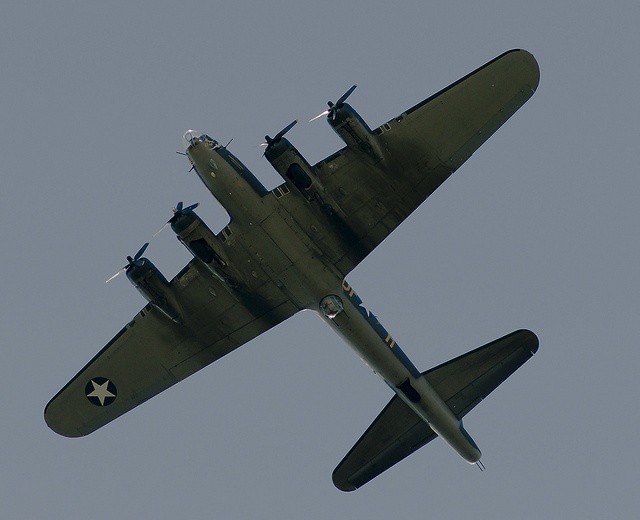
[44,48,540,492]
